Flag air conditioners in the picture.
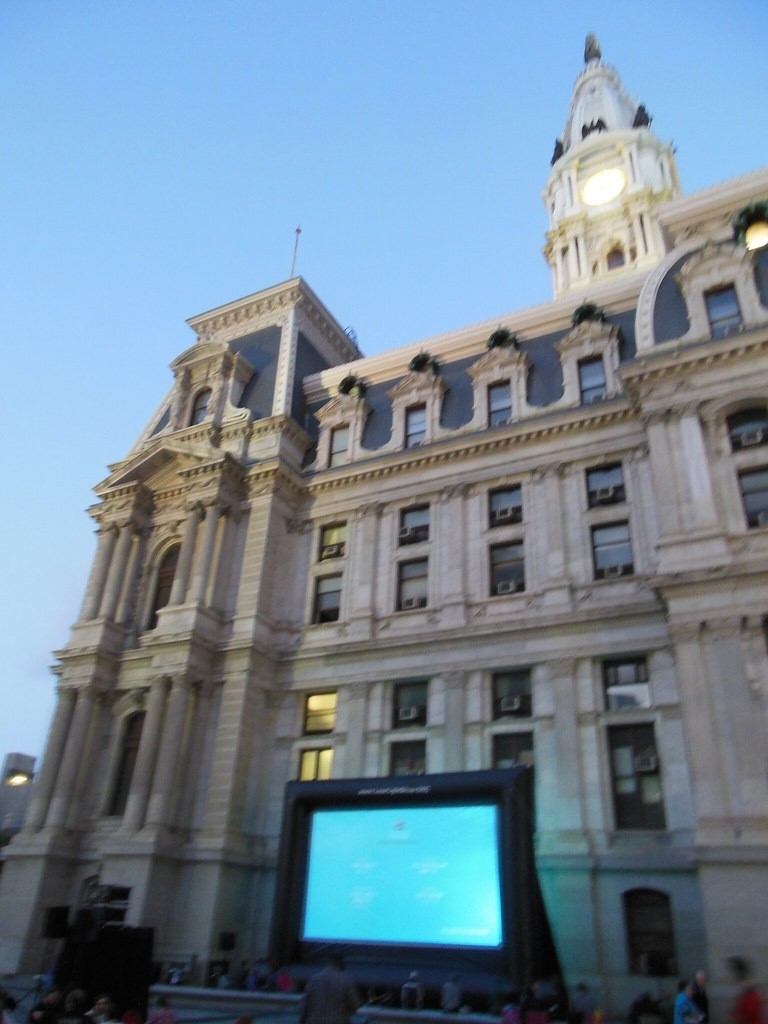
[496,416,522,426]
[496,506,513,520]
[497,580,514,593]
[398,707,415,720]
[402,596,419,609]
[399,527,415,539]
[592,391,616,404]
[741,429,762,445]
[604,563,623,577]
[596,485,614,500]
[411,439,432,448]
[501,696,520,711]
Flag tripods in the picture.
[15,940,52,1010]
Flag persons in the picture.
[441,974,462,1013]
[401,970,422,1010]
[673,967,709,1024]
[300,952,363,1024]
[726,957,768,1024]
[166,959,293,993]
[0,989,177,1024]
[503,981,589,1024]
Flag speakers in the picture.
[219,932,235,951]
[43,906,69,939]
